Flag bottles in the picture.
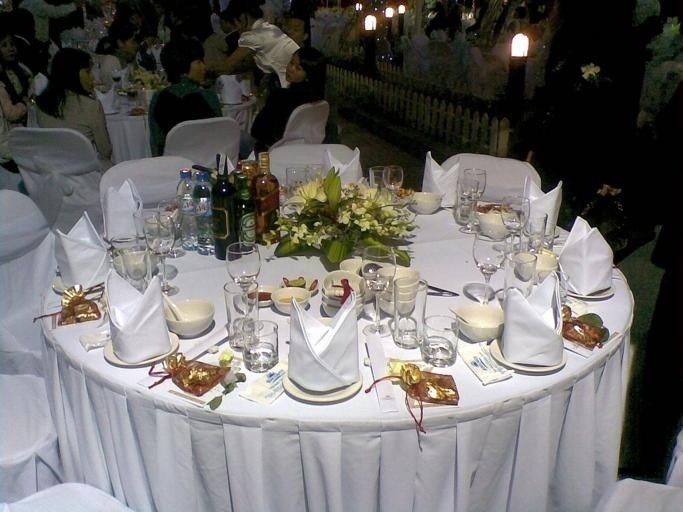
[176,153,281,261]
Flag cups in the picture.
[241,80,250,96]
[392,278,427,349]
[502,252,537,302]
[223,283,257,351]
[243,320,279,372]
[133,210,160,245]
[421,315,460,366]
[368,166,383,190]
[454,179,479,224]
[520,210,547,253]
[285,166,307,186]
[305,165,326,184]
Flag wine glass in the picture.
[474,230,508,304]
[361,245,396,338]
[127,87,139,110]
[111,69,123,90]
[459,168,487,234]
[143,215,176,292]
[501,196,531,250]
[383,167,404,192]
[226,242,261,291]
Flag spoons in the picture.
[161,292,193,322]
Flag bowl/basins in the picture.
[279,277,319,297]
[246,283,275,306]
[161,300,215,338]
[321,269,365,319]
[374,266,419,317]
[411,193,441,215]
[455,304,505,342]
[270,286,311,315]
[339,258,375,302]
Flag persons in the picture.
[1,2,327,161]
[595,86,683,479]
[507,6,528,34]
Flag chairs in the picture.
[6,127,100,230]
[1,484,134,512]
[160,117,238,172]
[269,100,363,190]
[1,373,64,501]
[100,156,196,239]
[443,154,542,207]
[597,430,683,511]
[0,189,49,377]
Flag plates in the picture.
[220,101,243,105]
[564,286,614,299]
[104,109,119,115]
[489,338,567,372]
[279,372,363,403]
[105,331,181,367]
[54,274,106,293]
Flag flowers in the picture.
[272,169,421,266]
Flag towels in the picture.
[323,146,362,189]
[557,216,614,296]
[421,151,462,208]
[55,210,109,291]
[521,175,563,240]
[500,269,563,366]
[288,291,359,393]
[104,178,144,241]
[104,270,170,366]
